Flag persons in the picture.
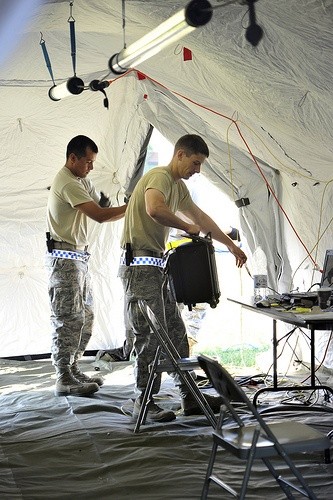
[114,133,250,426]
[44,134,128,398]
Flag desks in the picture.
[227,296,333,389]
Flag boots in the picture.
[55,369,100,397]
[132,392,176,422]
[180,385,223,415]
[69,366,104,387]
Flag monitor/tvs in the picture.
[320,250,333,289]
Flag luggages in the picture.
[168,231,221,311]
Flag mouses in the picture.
[256,300,271,308]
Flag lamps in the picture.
[107,0,214,75]
[39,0,86,102]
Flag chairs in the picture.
[196,355,333,500]
[133,299,218,435]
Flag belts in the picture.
[45,248,90,262]
[121,256,164,268]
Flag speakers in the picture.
[165,237,223,311]
